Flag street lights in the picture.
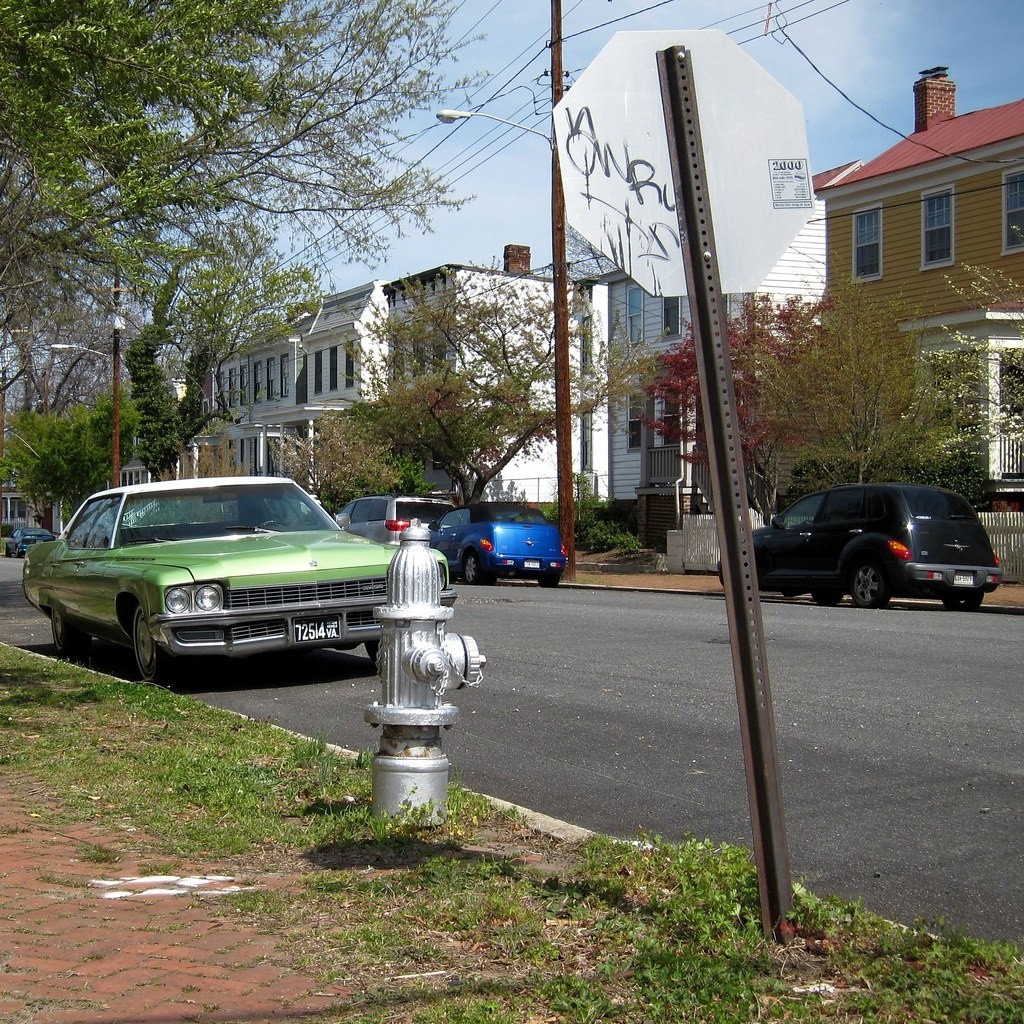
[436,110,577,578]
[50,344,119,487]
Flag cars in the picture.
[21,477,458,686]
[717,483,1003,611]
[428,502,568,588]
[5,528,55,558]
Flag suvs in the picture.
[330,493,464,548]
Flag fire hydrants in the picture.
[365,517,486,827]
